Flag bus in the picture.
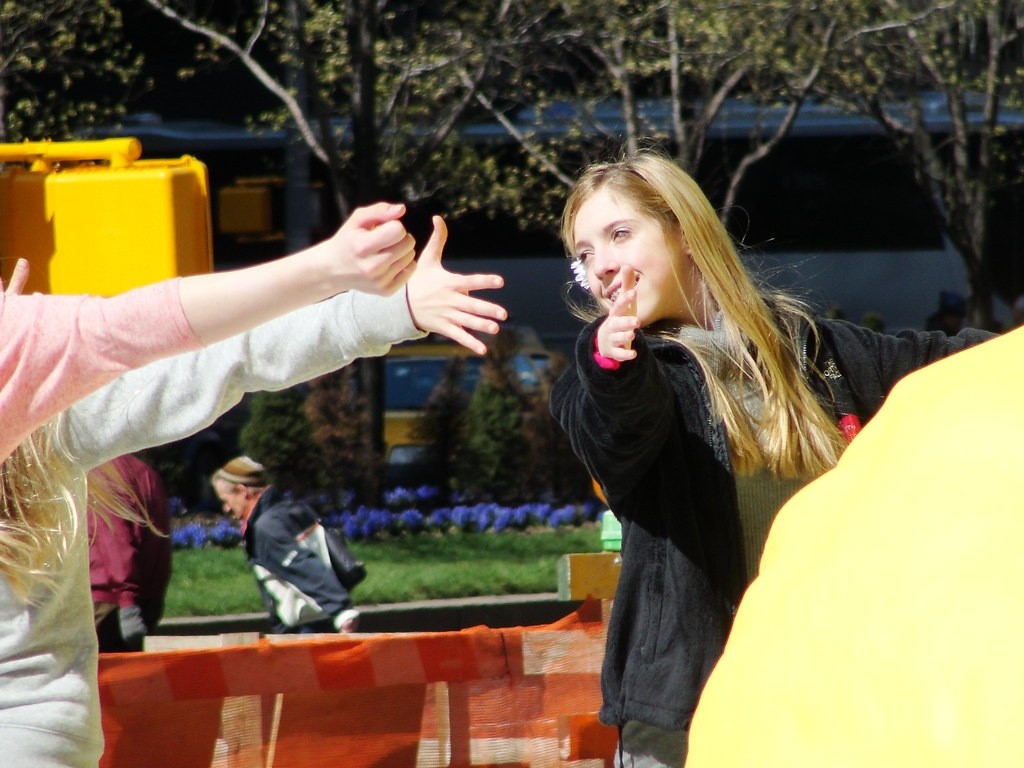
[120,89,1023,464]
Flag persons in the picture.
[549,153,1023,768]
[212,456,360,635]
[685,324,1024,768]
[0,202,506,768]
[87,454,172,653]
